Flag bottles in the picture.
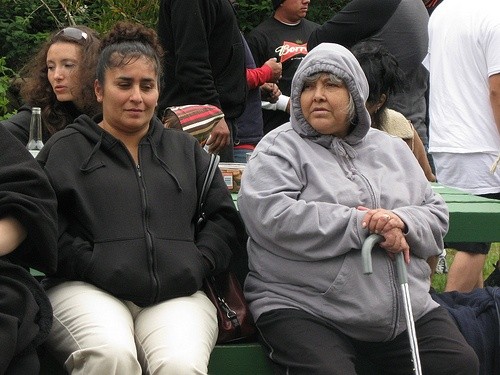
[25,108,45,159]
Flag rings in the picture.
[384,214,390,222]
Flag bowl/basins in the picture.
[219,161,248,194]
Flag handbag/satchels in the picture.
[196,154,256,343]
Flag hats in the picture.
[274,0,285,11]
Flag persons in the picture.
[0,24,103,151]
[245,0,324,134]
[346,41,435,182]
[31,24,242,375]
[417,0,500,294]
[0,121,62,375]
[154,0,264,166]
[305,0,438,183]
[160,103,225,146]
[233,42,482,374]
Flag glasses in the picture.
[49,27,88,42]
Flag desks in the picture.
[231,181,500,242]
